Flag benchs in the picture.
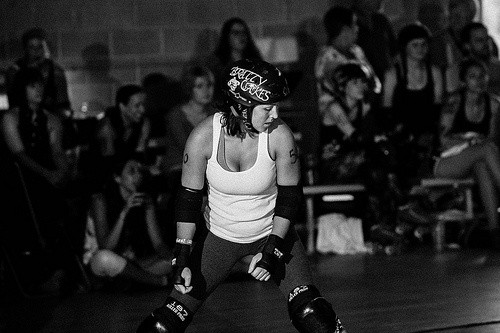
[301,177,475,254]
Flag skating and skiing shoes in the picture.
[395,201,434,238]
[370,225,409,256]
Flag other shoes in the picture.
[156,246,174,270]
[143,272,165,290]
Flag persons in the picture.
[0,0,500,288]
[135,58,348,333]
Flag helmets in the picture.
[333,63,366,84]
[400,24,431,48]
[221,58,291,108]
